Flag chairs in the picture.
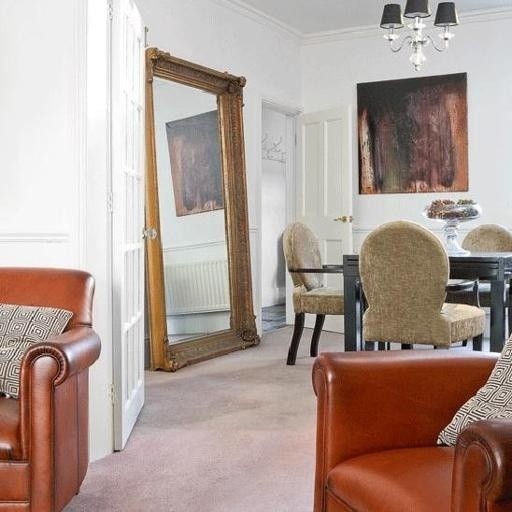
[0,265,105,512]
[454,225,512,351]
[283,221,364,365]
[311,346,512,512]
[357,219,484,355]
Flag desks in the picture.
[338,251,510,354]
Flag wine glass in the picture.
[421,204,483,256]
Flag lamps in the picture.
[377,1,461,74]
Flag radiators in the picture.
[162,259,231,318]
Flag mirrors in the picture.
[146,47,260,372]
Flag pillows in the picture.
[0,297,75,400]
[437,335,512,445]
